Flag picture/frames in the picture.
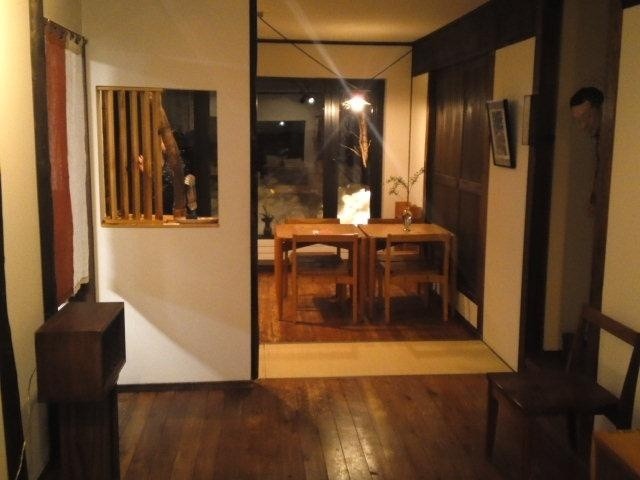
[485,97,514,168]
[521,95,531,146]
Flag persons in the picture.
[260,127,293,163]
[570,88,604,377]
[137,125,200,215]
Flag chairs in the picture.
[484,302,639,478]
[273,218,456,323]
[587,428,640,479]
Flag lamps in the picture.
[258,17,453,110]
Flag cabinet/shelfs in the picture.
[33,299,128,479]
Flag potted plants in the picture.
[260,202,275,241]
[385,165,429,231]
